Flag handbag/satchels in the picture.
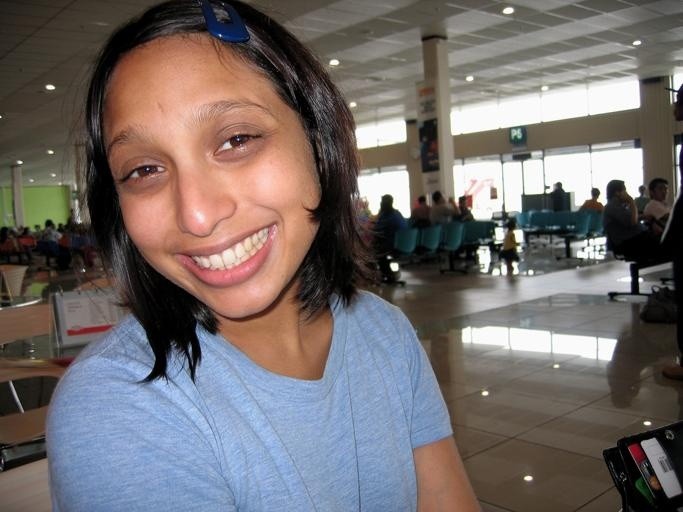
[639,285,679,325]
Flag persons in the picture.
[577,186,602,213]
[634,183,646,213]
[42,0,482,511]
[498,219,519,273]
[358,181,478,258]
[550,180,569,212]
[658,83,682,385]
[608,331,668,410]
[1,208,101,279]
[603,175,673,264]
[645,177,671,227]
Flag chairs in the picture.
[386,207,604,287]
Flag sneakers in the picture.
[663,366,683,380]
